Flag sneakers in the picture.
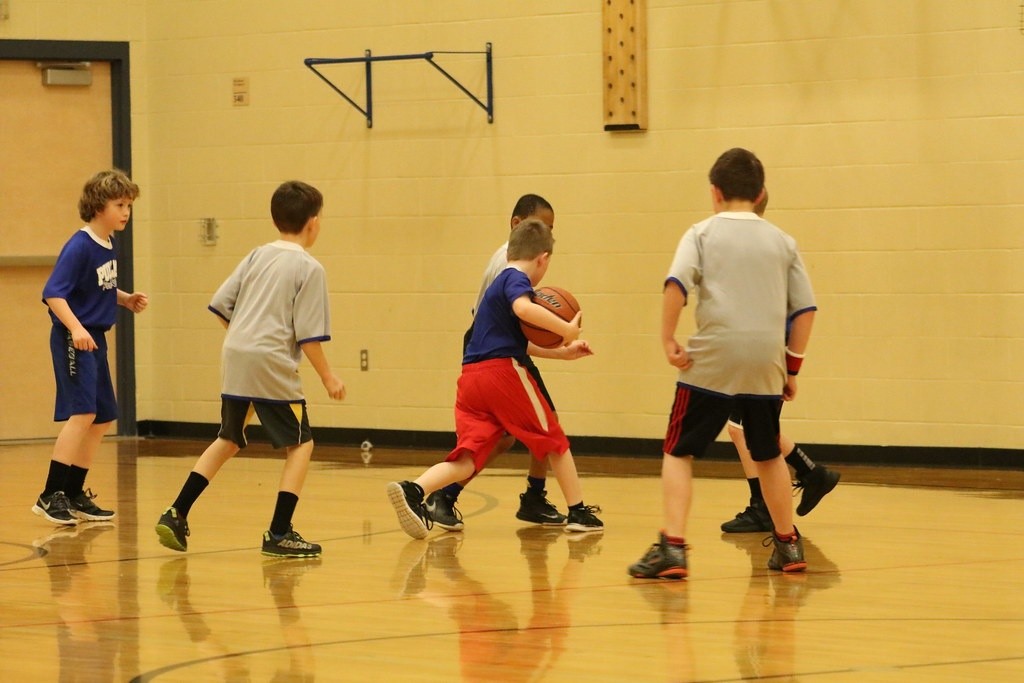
[261,522,321,557]
[566,505,604,531]
[791,464,840,516]
[155,507,190,552]
[421,491,464,530]
[720,506,775,532]
[626,531,689,577]
[32,491,78,526]
[516,490,568,525]
[762,525,807,572]
[387,480,433,539]
[68,488,115,521]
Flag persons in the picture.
[388,195,605,539]
[32,170,148,524]
[629,148,840,577]
[155,180,346,556]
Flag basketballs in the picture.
[520,285,582,349]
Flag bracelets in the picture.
[783,348,805,376]
